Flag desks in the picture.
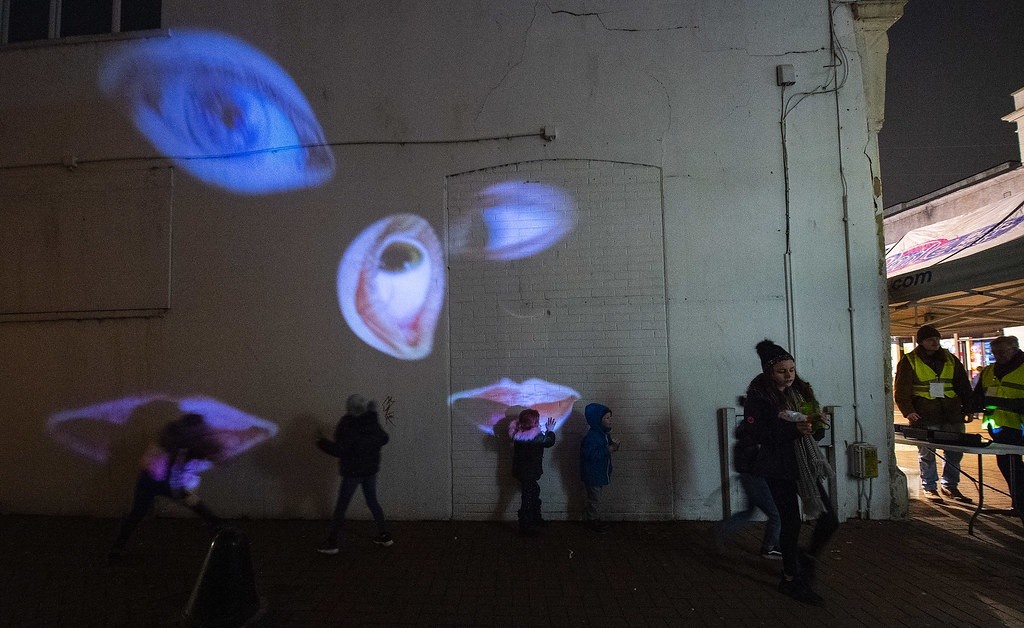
[894,439,1024,535]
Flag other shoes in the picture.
[317,542,339,555]
[779,576,824,606]
[924,489,943,503]
[588,520,609,535]
[372,535,393,547]
[941,486,972,502]
[762,546,783,561]
[799,547,816,583]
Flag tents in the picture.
[885,191,1024,382]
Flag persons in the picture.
[111,413,236,559]
[966,336,1024,517]
[316,395,394,553]
[508,409,557,537]
[894,326,972,504]
[579,403,620,535]
[711,341,841,606]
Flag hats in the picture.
[917,325,941,344]
[756,339,794,373]
